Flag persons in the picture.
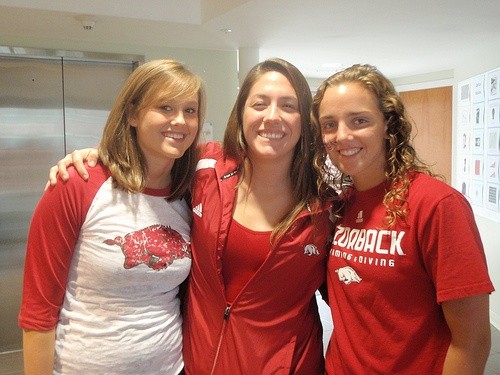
[307,61,495,375]
[16,60,194,375]
[42,56,342,375]
